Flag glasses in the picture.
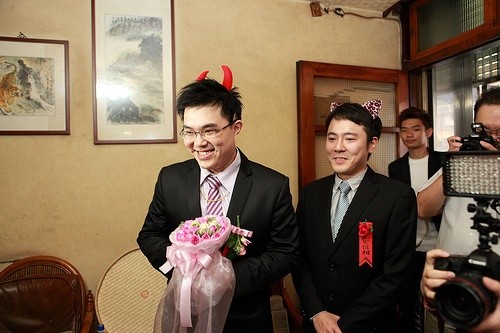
[178,121,233,141]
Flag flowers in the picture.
[228,215,248,256]
[169,214,234,253]
[358,219,373,239]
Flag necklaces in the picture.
[199,189,229,201]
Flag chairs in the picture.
[0,256,94,333]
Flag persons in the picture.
[290,102,418,333]
[136,79,300,333]
[417,86,500,333]
[419,249,500,333]
[387,106,447,333]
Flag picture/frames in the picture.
[0,36,71,135]
[91,0,178,144]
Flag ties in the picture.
[333,180,351,244]
[205,174,223,216]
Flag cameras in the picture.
[430,122,500,329]
